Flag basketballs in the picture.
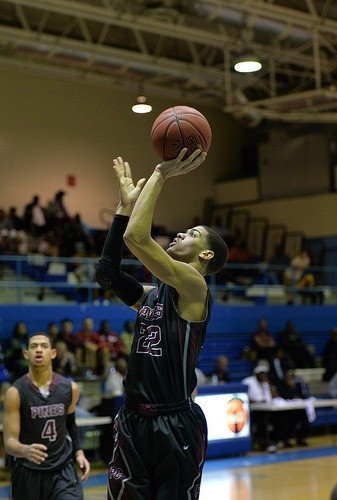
[149,105,212,163]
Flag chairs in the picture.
[0,227,337,304]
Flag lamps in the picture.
[234,42,262,73]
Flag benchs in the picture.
[0,331,337,382]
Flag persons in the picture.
[0,190,324,307]
[2,332,91,500]
[2,319,337,452]
[94,106,229,500]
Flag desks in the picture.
[0,417,112,471]
[251,399,337,448]
